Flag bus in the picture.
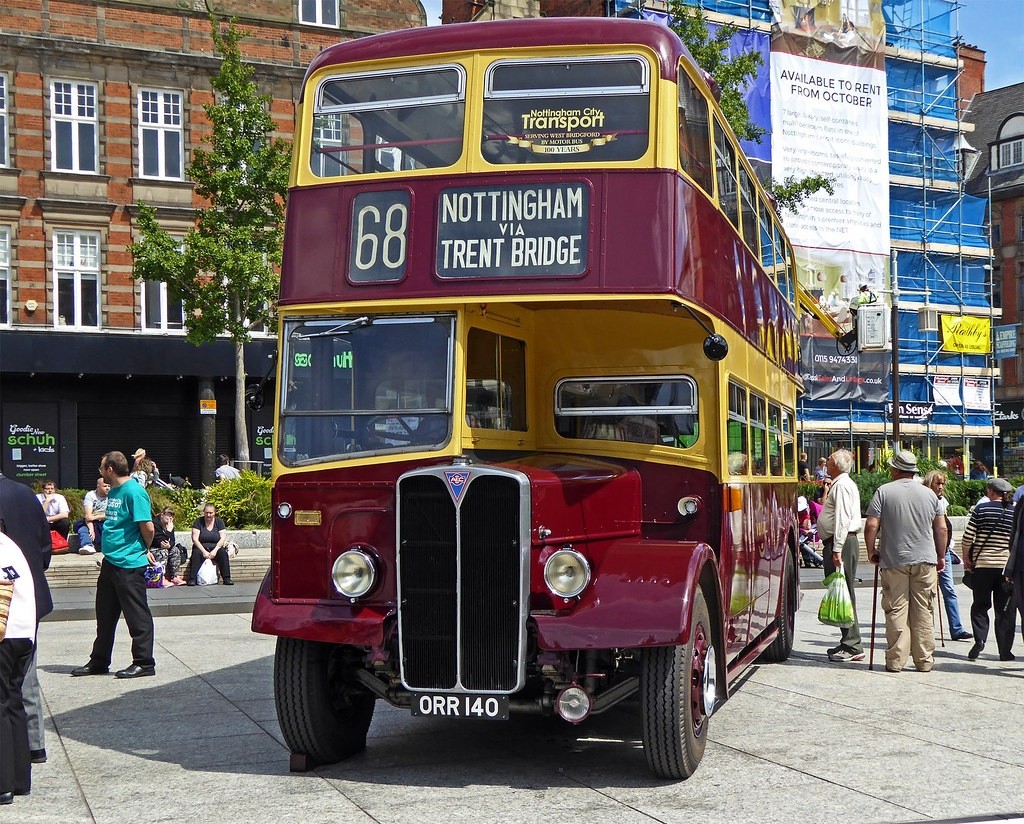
[250,16,803,779]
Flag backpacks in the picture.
[50,530,69,553]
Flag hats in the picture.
[988,478,1012,493]
[798,496,807,511]
[889,451,920,472]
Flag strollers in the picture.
[799,524,825,569]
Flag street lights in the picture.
[852,248,938,459]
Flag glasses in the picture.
[98,466,115,473]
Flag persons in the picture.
[623,419,783,478]
[800,449,1024,672]
[0,449,243,803]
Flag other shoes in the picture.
[163,579,174,588]
[223,578,234,585]
[188,579,196,585]
[952,631,973,640]
[171,576,187,585]
[12,788,30,794]
[0,792,14,804]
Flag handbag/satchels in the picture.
[145,566,163,588]
[963,571,973,590]
[196,559,218,585]
[222,541,239,558]
[175,543,187,564]
[0,580,15,643]
[818,562,855,628]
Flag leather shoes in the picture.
[968,640,984,658]
[1000,651,1015,661]
[115,664,155,678]
[70,660,110,676]
[31,748,47,764]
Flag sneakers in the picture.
[829,649,865,662]
[79,545,96,554]
[827,645,844,655]
